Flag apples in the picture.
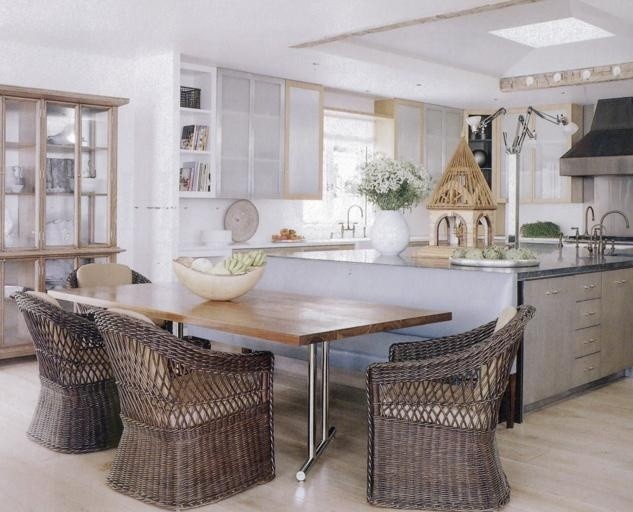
[178,256,232,275]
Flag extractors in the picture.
[559,96,633,177]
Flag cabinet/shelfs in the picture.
[523,268,633,409]
[218,67,285,199]
[285,79,324,200]
[0,85,130,362]
[375,100,424,180]
[423,103,468,197]
[179,63,216,198]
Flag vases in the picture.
[369,210,410,256]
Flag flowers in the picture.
[354,156,432,210]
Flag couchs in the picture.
[66,264,152,289]
[366,304,536,512]
[95,307,276,512]
[14,290,124,454]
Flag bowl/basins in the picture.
[68,177,100,194]
[170,256,267,301]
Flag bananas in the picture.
[221,249,267,274]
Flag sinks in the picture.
[570,234,633,243]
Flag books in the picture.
[179,160,211,192]
[180,124,209,151]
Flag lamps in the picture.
[466,116,486,137]
[560,114,579,136]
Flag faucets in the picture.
[591,223,608,241]
[600,210,630,242]
[585,205,595,236]
[346,204,364,229]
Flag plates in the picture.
[275,240,305,243]
[223,199,260,243]
[200,231,232,247]
